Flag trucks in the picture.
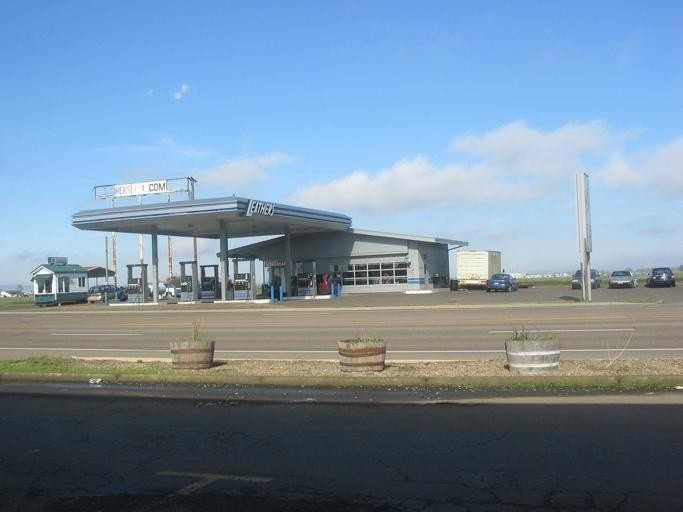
[456,250,502,290]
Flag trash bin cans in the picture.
[450,280,458,291]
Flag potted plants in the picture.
[503,319,562,374]
[168,316,216,371]
[337,335,386,372]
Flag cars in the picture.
[607,271,636,289]
[486,273,519,293]
[119,282,201,299]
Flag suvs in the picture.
[86,284,120,303]
[647,267,675,288]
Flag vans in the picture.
[571,269,601,289]
